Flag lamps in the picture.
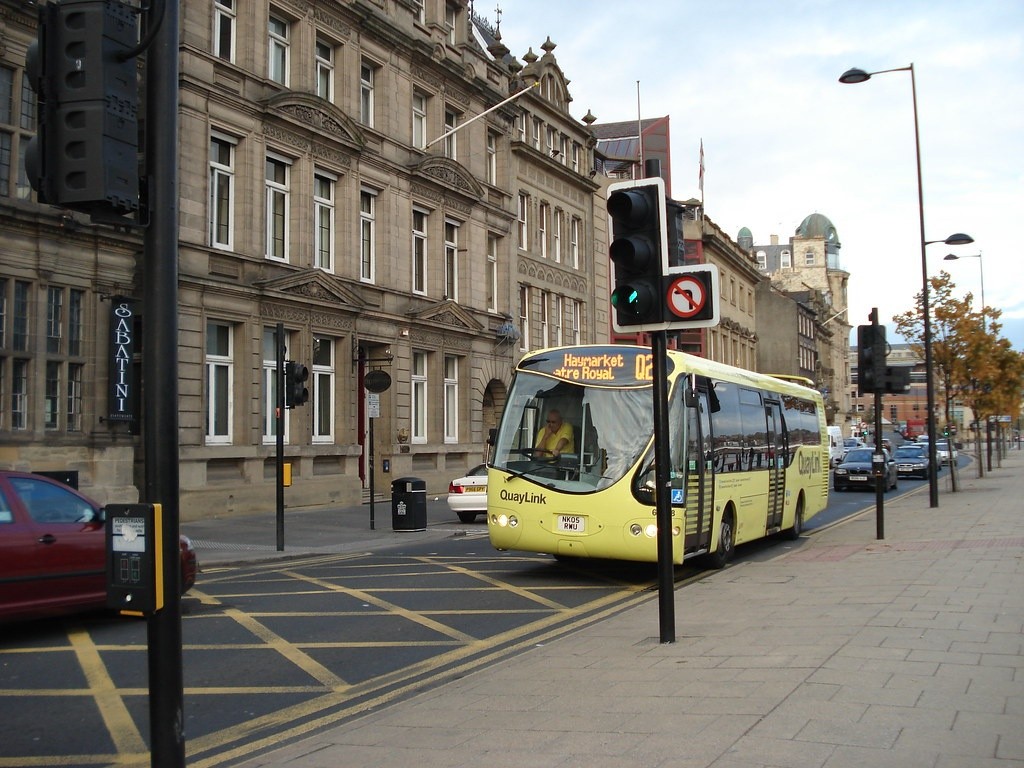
[312,336,320,343]
[57,213,74,231]
[399,326,409,338]
[385,348,391,355]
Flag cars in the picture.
[834,448,898,491]
[844,439,867,454]
[892,446,930,479]
[0,469,197,634]
[892,417,961,470]
[881,439,893,451]
[448,464,494,523]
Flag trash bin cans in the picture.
[391,477,427,533]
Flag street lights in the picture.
[838,63,978,510]
[942,253,988,334]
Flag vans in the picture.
[827,425,846,469]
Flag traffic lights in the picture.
[605,176,677,334]
[103,503,165,617]
[282,360,309,409]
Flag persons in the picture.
[533,410,574,463]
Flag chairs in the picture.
[573,426,581,454]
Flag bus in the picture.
[483,344,833,562]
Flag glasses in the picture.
[547,420,560,423]
